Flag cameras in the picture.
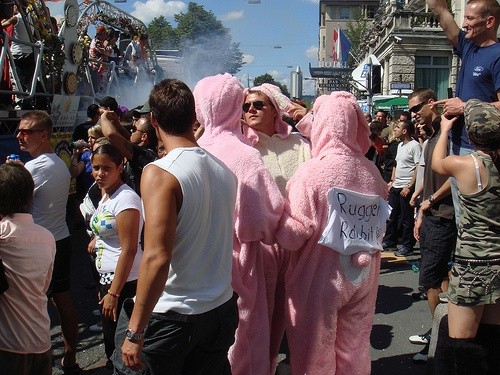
[98,107,114,119]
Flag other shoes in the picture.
[412,287,428,300]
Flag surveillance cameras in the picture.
[395,36,403,41]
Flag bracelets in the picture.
[107,289,120,299]
[407,184,411,189]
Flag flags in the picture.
[332,29,351,63]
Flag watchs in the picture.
[428,195,434,203]
[125,325,149,342]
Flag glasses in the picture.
[130,126,145,133]
[408,101,427,113]
[242,101,265,113]
[15,128,46,136]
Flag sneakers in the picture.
[381,241,414,257]
[408,328,432,362]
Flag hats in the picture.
[95,96,118,111]
[463,98,500,144]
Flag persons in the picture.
[363,107,429,183]
[192,69,287,375]
[429,98,500,375]
[406,88,457,346]
[381,119,424,256]
[425,0,499,302]
[275,90,391,375]
[111,77,242,375]
[234,84,319,375]
[6,108,82,375]
[0,162,56,375]
[77,132,138,307]
[87,141,147,375]
[0,0,204,197]
